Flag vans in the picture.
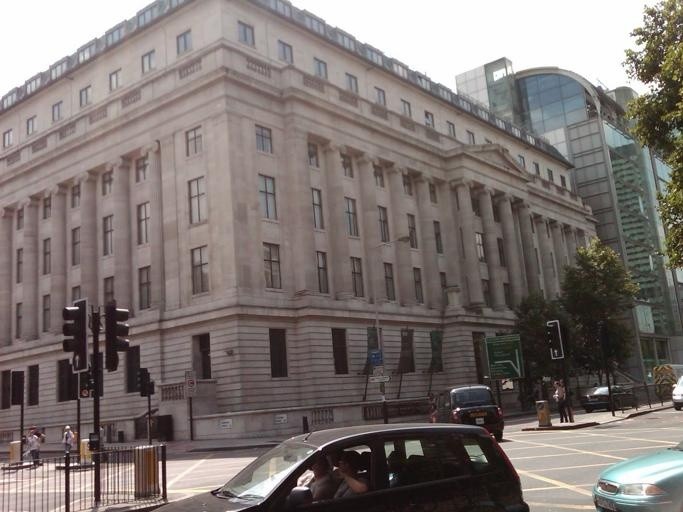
[432,385,504,442]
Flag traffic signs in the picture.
[369,376,391,383]
[484,334,527,381]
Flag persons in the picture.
[296,469,314,487]
[593,383,598,387]
[560,379,574,422]
[283,453,301,467]
[25,431,40,469]
[334,451,368,498]
[305,456,334,499]
[28,426,45,466]
[63,426,74,458]
[553,381,568,423]
[531,377,546,400]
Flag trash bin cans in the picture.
[134,445,160,499]
[81,439,92,465]
[9,441,21,465]
[535,400,552,427]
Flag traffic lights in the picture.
[61,306,83,354]
[115,307,130,352]
[136,370,143,389]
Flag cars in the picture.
[671,374,683,410]
[582,385,639,411]
[591,441,683,511]
[147,423,530,512]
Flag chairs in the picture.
[331,449,478,486]
